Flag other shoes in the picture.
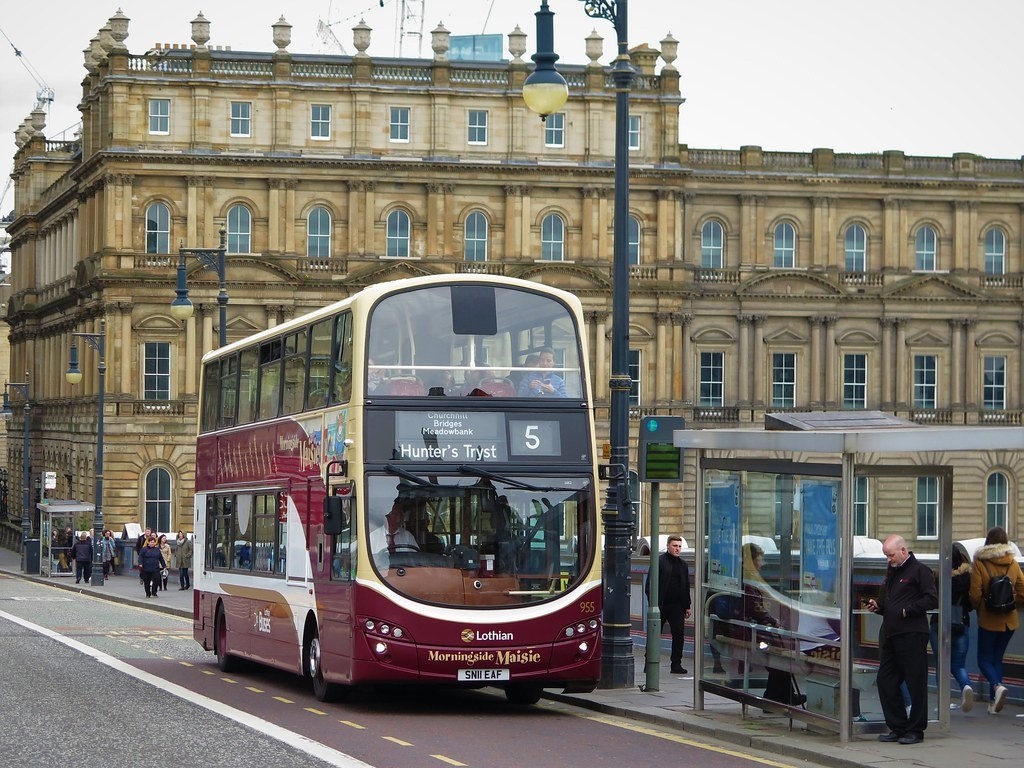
[671,663,687,673]
[713,667,726,674]
[897,730,924,743]
[852,713,871,722]
[879,731,902,742]
[961,685,974,712]
[987,703,998,714]
[739,665,754,674]
[789,693,807,706]
[987,686,1008,712]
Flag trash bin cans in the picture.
[24,539,40,574]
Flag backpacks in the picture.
[951,593,965,637]
[978,558,1016,614]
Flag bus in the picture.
[193,273,633,707]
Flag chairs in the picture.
[307,374,515,408]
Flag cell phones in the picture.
[861,599,878,610]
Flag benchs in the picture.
[708,614,880,677]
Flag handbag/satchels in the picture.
[161,568,169,579]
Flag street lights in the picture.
[174,224,230,351]
[519,1,664,693]
[0,469,8,520]
[0,371,34,572]
[63,316,104,587]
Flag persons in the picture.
[930,542,973,714]
[441,365,495,395]
[240,542,251,567]
[488,513,514,542]
[518,348,569,398]
[371,502,420,553]
[52,527,73,559]
[136,527,171,597]
[272,361,297,416]
[70,527,118,583]
[174,530,193,591]
[798,613,867,722]
[410,510,441,544]
[368,361,382,391]
[506,355,539,386]
[733,544,807,707]
[863,534,938,744]
[968,526,1024,716]
[645,535,692,674]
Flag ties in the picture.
[388,534,395,553]
